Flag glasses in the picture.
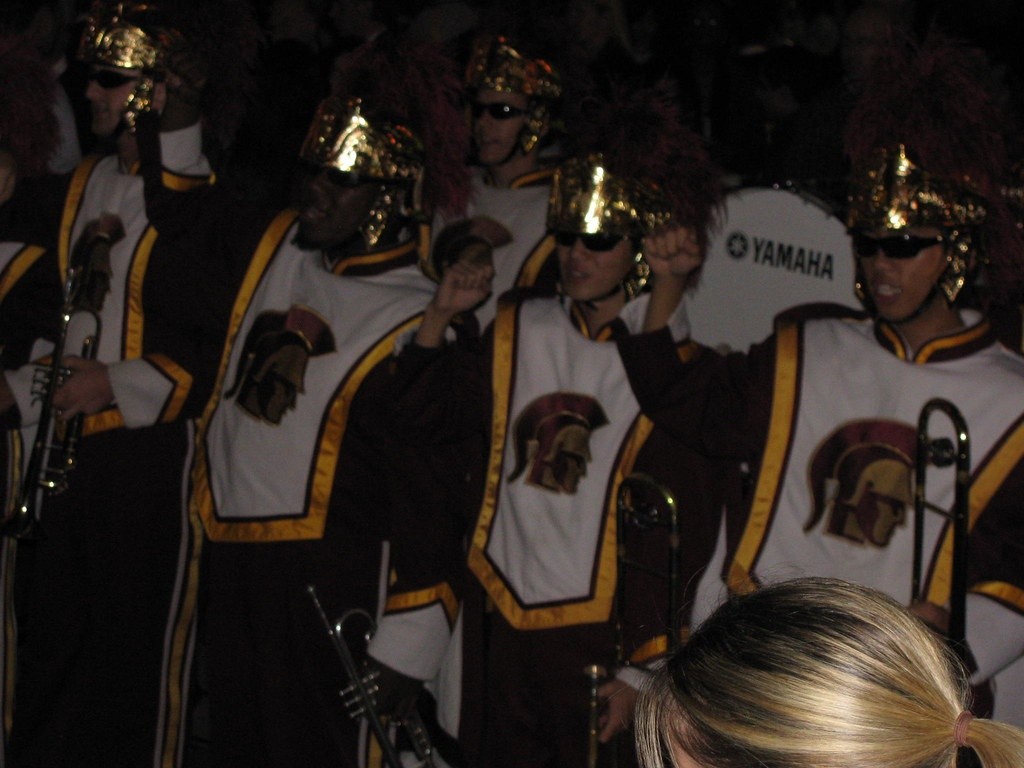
[303,158,369,189]
[554,229,624,253]
[851,232,944,260]
[470,99,530,119]
[75,64,144,89]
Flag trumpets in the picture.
[310,586,434,768]
[584,619,633,768]
[911,399,970,645]
[0,261,101,541]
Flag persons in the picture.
[0,0,1024,768]
